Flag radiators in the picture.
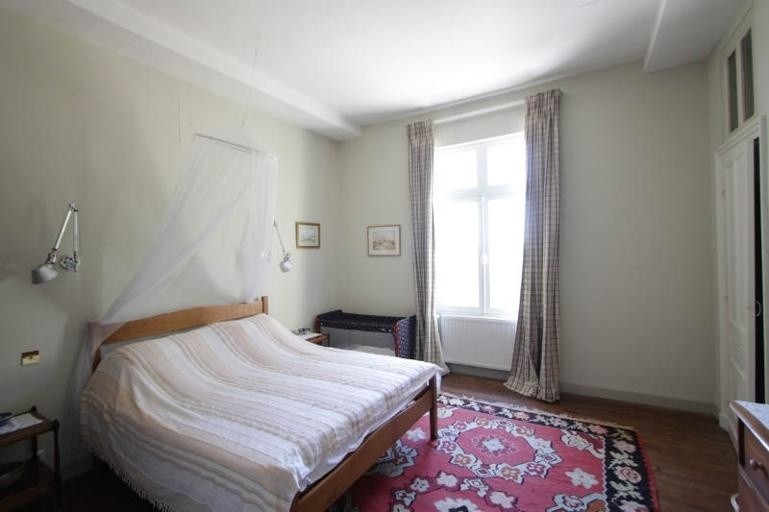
[441,316,517,372]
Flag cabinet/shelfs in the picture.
[730,400,769,512]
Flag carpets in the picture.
[327,390,659,512]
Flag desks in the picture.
[0,407,62,512]
[315,310,417,360]
[294,331,329,346]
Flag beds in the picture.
[78,295,441,512]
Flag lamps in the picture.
[31,200,79,285]
[273,221,292,273]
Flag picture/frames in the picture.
[366,225,401,256]
[296,222,321,247]
